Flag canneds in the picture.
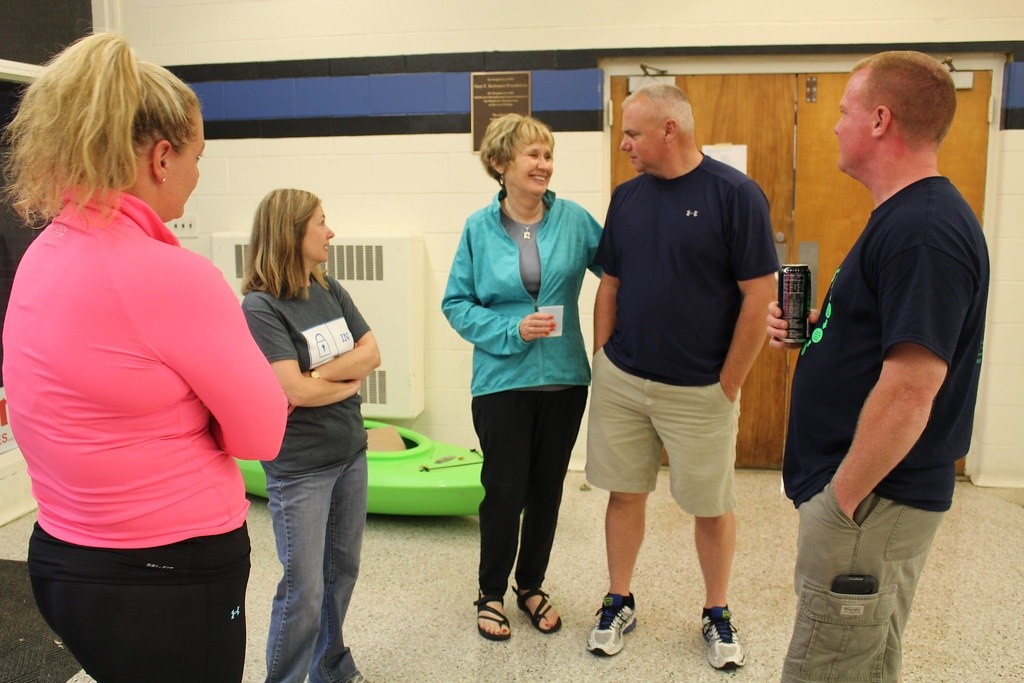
[778,264,812,343]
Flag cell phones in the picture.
[831,574,877,595]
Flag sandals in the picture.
[512,585,561,634]
[474,589,511,640]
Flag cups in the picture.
[537,305,564,338]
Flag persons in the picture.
[441,113,604,640]
[586,84,780,669]
[2,34,288,683]
[242,189,381,683]
[765,50,990,683]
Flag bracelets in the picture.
[308,368,320,379]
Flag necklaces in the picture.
[505,200,542,238]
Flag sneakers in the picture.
[588,594,637,657]
[701,606,746,669]
